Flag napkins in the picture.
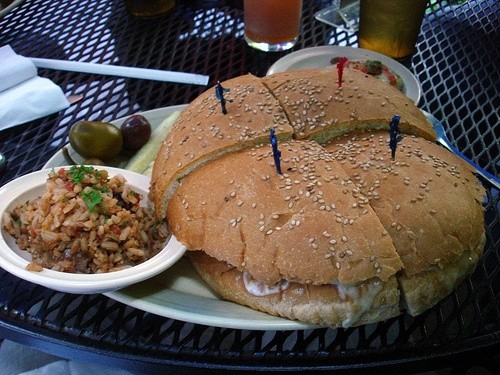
[0,45,69,131]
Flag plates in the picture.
[0,166,186,294]
[266,45,421,107]
[42,104,322,328]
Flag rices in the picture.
[4,165,165,275]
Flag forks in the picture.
[418,108,500,203]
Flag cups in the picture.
[357,0,426,64]
[243,0,303,53]
[126,0,175,20]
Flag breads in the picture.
[149,64,486,325]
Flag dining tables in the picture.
[0,0,500,375]
